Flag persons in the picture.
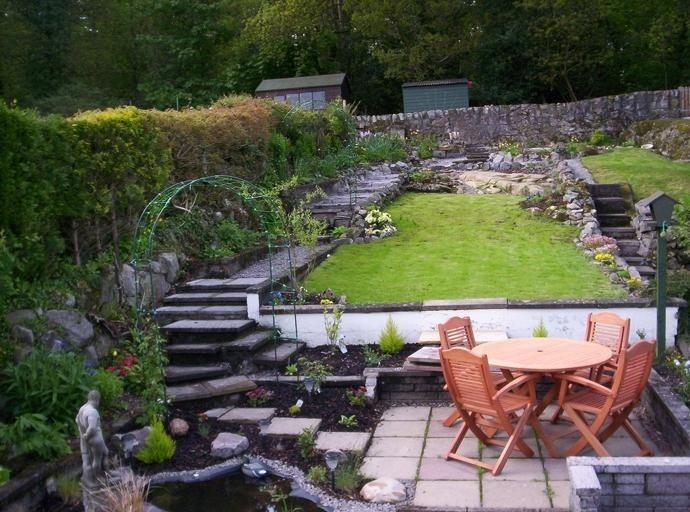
[75,390,118,480]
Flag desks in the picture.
[469,336,614,461]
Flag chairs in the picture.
[561,335,659,465]
[549,309,633,428]
[436,314,540,437]
[437,343,539,475]
